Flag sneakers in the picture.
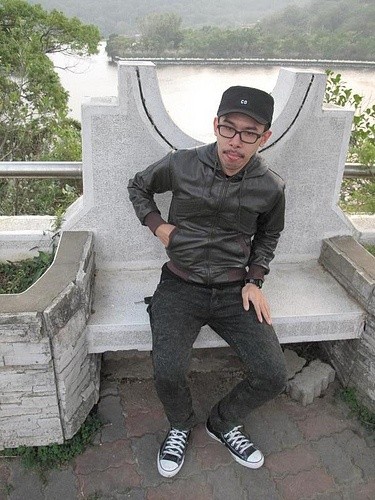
[204,416,265,469]
[157,425,193,478]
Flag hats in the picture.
[217,87,274,125]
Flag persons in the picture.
[127,86,290,478]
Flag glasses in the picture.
[216,117,267,144]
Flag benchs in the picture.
[61,61,367,354]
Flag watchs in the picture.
[246,279,263,289]
